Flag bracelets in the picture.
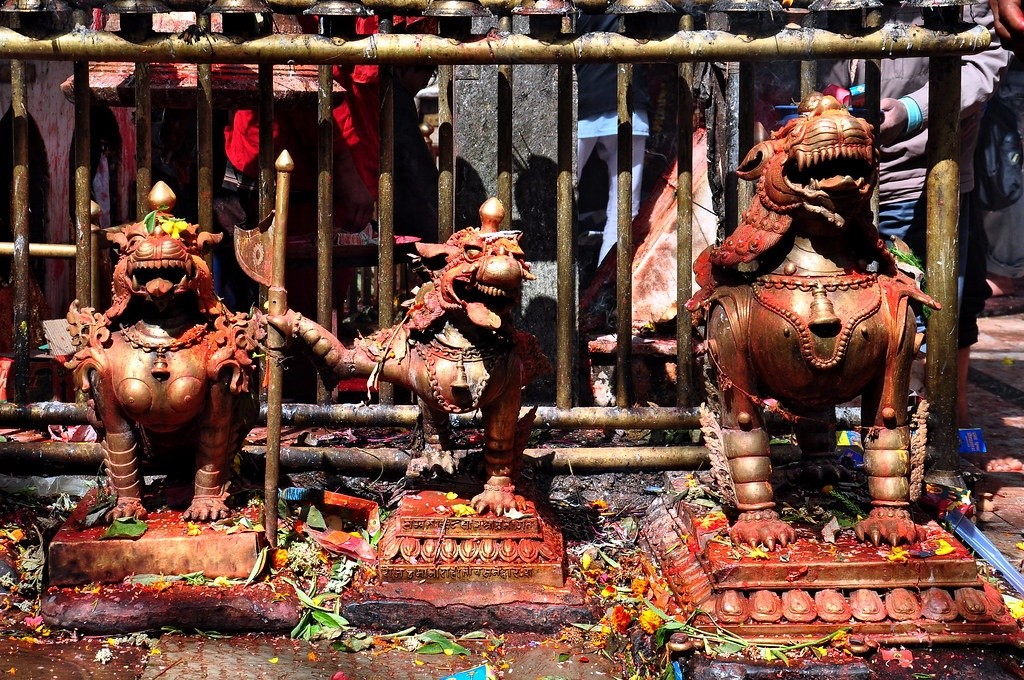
[896,95,922,133]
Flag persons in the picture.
[565,12,651,267]
[805,0,1024,434]
[376,46,450,278]
